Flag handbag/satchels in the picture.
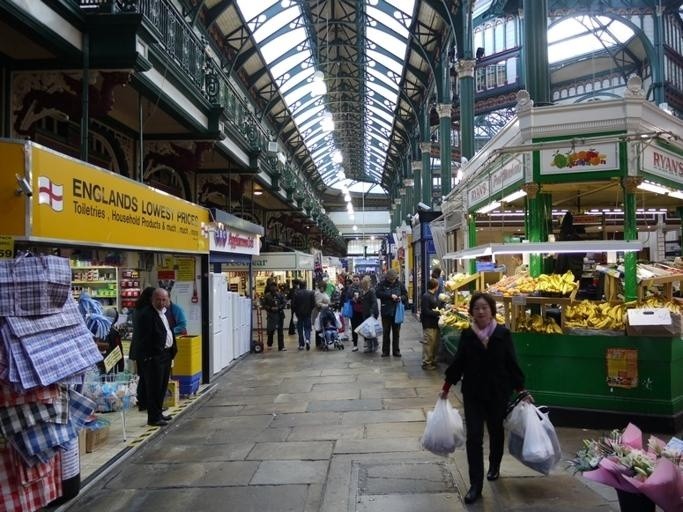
[342,299,354,318]
[0,385,98,468]
[0,255,72,317]
[0,293,104,394]
[0,444,64,512]
[394,301,405,324]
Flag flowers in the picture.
[562,423,683,511]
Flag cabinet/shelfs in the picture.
[440,261,683,433]
[70,266,141,313]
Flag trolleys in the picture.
[252,304,264,353]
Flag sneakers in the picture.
[306,340,310,351]
[352,347,358,351]
[296,345,304,350]
[393,352,401,357]
[423,363,438,370]
[381,352,389,357]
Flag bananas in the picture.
[507,270,578,295]
[514,313,564,335]
[637,297,683,316]
[565,300,627,332]
[443,314,469,329]
[496,313,505,325]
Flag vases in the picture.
[614,487,657,511]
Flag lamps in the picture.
[440,154,491,258]
[491,130,643,254]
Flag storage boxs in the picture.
[78,429,87,457]
[625,308,673,335]
[87,426,110,452]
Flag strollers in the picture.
[316,304,344,351]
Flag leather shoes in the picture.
[486,466,500,480]
[148,419,167,426]
[464,489,482,503]
[160,416,172,420]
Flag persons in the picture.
[421,268,443,301]
[139,287,178,425]
[439,293,534,504]
[129,287,167,412]
[163,292,187,336]
[263,270,408,356]
[421,279,440,370]
[511,254,526,275]
